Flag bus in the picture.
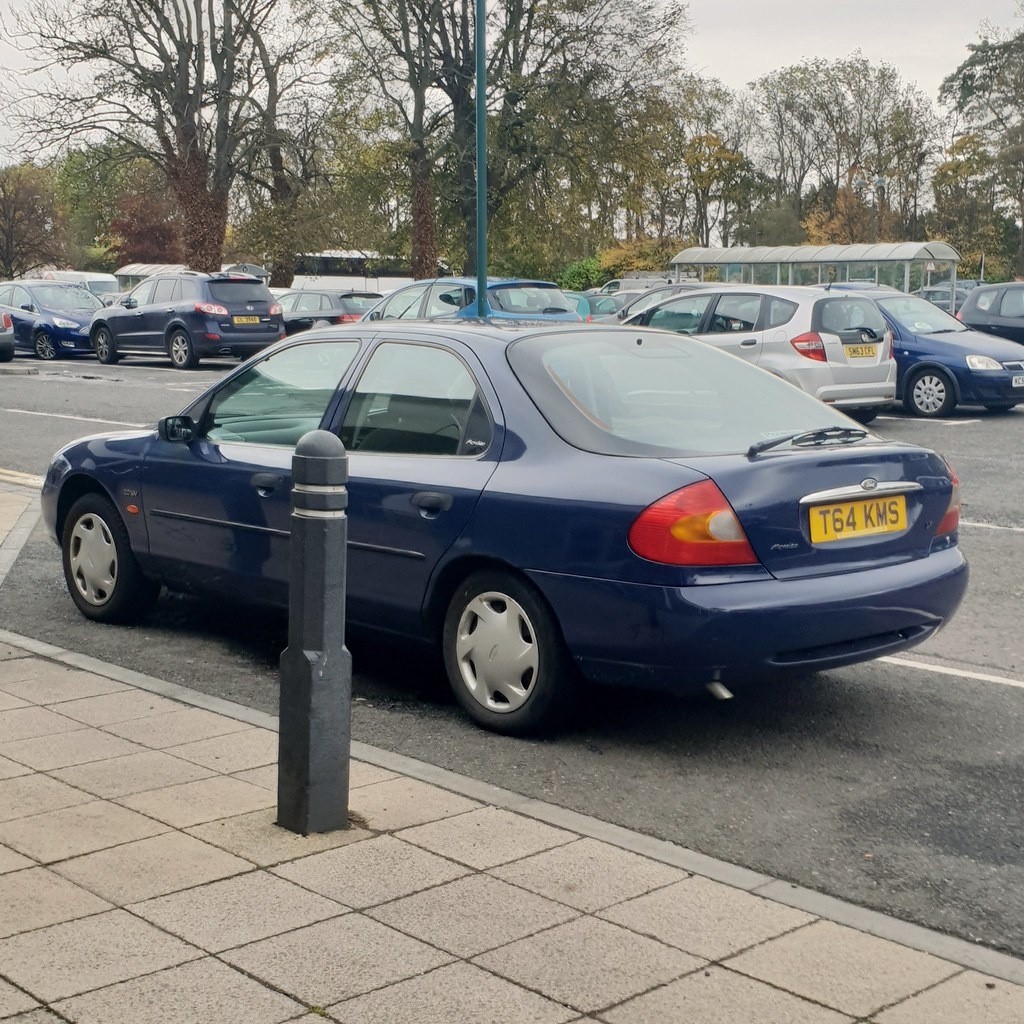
[294,250,461,295]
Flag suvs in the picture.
[89,270,288,369]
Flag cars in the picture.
[0,308,15,364]
[357,274,583,325]
[40,317,970,744]
[0,280,125,361]
[560,278,1024,425]
[276,288,383,336]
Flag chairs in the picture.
[356,380,461,453]
